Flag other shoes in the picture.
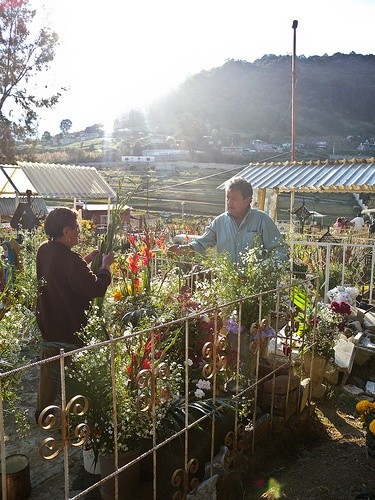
[35,409,55,425]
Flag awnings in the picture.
[217,157,374,196]
[0,163,46,199]
[16,160,118,201]
[83,204,134,211]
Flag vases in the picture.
[96,439,145,500]
[303,350,330,399]
[366,443,375,470]
[82,433,100,474]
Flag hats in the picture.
[44,207,77,235]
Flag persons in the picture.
[34,206,118,426]
[333,213,375,236]
[165,177,291,391]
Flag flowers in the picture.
[0,220,375,500]
